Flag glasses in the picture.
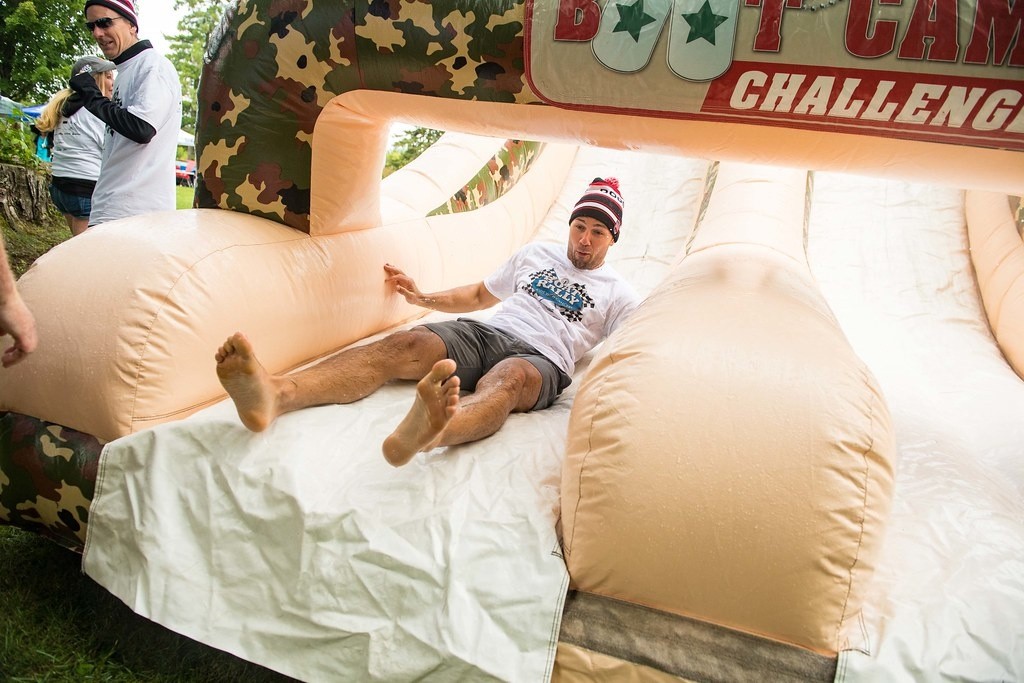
[85,16,123,31]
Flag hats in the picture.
[568,176,624,244]
[72,54,117,78]
[84,0,138,33]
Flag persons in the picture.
[72,1,183,228]
[0,232,38,372]
[35,57,118,243]
[209,173,644,468]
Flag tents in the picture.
[0,94,197,186]
